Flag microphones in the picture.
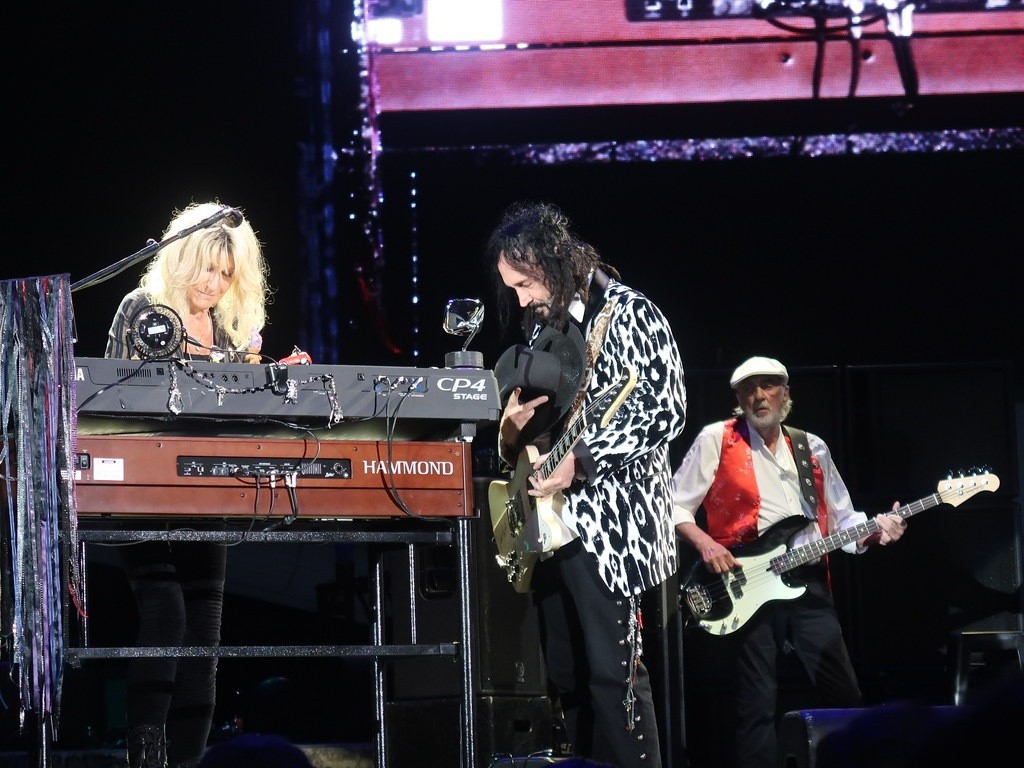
[223,208,243,229]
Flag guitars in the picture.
[485,362,642,598]
[678,468,1003,637]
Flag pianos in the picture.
[58,344,503,532]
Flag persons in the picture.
[665,358,906,768]
[107,201,315,768]
[492,203,688,768]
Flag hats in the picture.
[729,356,789,387]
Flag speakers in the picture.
[769,702,1024,768]
[384,476,555,768]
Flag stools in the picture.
[947,631,1024,706]
